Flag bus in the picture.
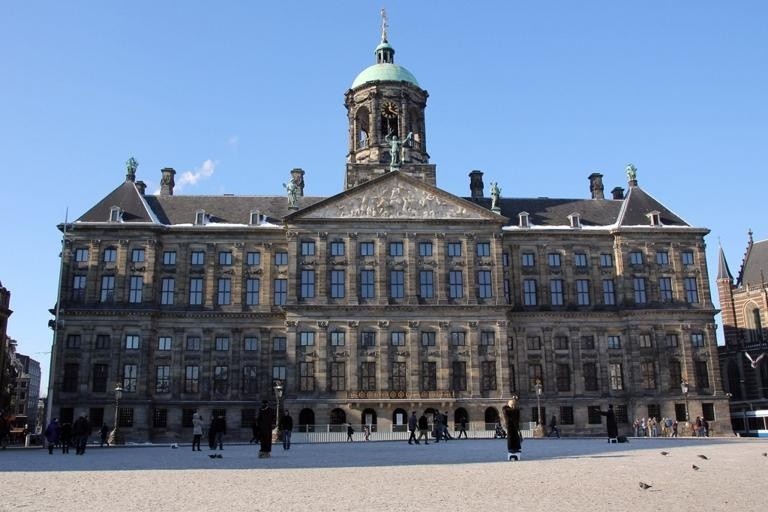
[729,399,768,437]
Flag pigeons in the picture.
[692,464,700,471]
[218,453,223,458]
[697,454,709,460]
[208,453,216,458]
[638,481,652,491]
[762,452,767,458]
[659,451,669,455]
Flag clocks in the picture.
[380,100,400,118]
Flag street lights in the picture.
[678,378,695,436]
[532,378,547,439]
[271,385,284,442]
[107,385,128,444]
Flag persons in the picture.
[385,131,412,167]
[347,423,353,442]
[494,416,507,437]
[547,415,560,439]
[250,400,292,458]
[407,412,468,445]
[490,181,501,208]
[192,411,226,458]
[634,415,709,437]
[44,416,92,455]
[593,404,618,438]
[99,422,110,448]
[502,399,521,452]
[364,426,370,440]
[287,178,298,205]
